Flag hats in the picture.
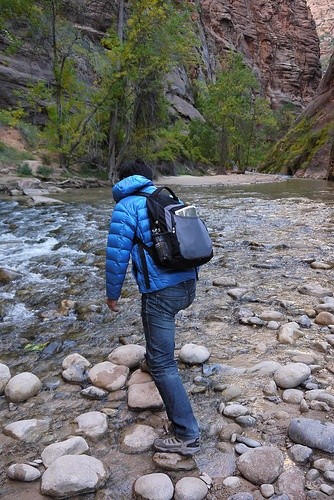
[118,160,153,179]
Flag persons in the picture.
[101,156,202,455]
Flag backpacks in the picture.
[127,186,214,270]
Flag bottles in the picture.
[151,222,171,261]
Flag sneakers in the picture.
[138,356,155,379]
[154,430,201,455]
